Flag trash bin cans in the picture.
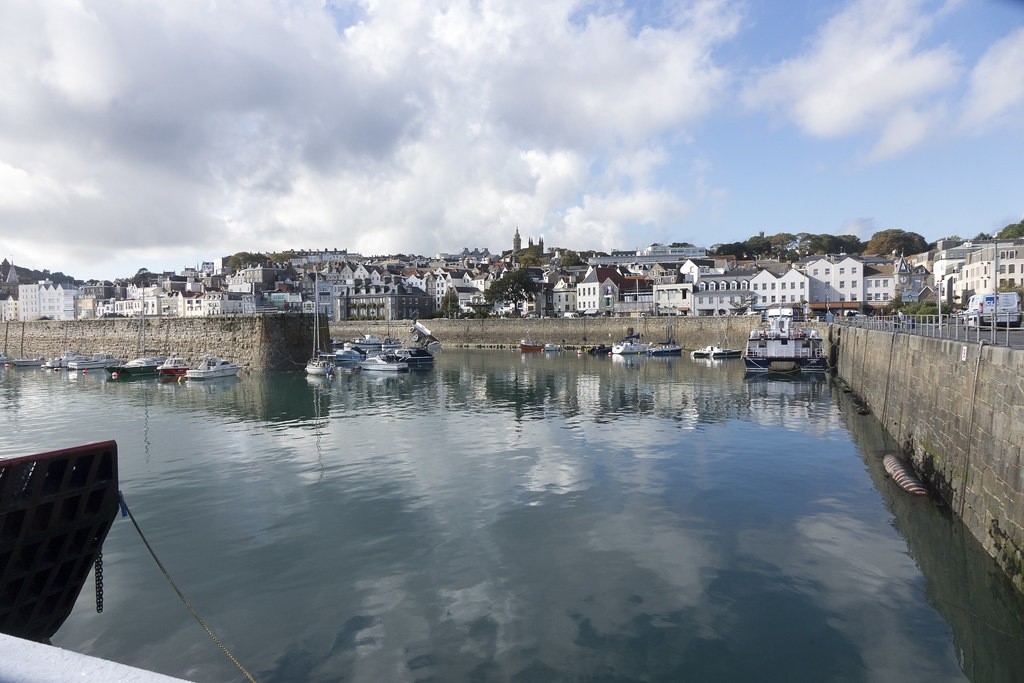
[605,310,611,317]
[908,319,915,329]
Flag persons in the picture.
[898,310,904,329]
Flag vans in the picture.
[961,292,1022,331]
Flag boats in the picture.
[186,358,242,378]
[156,357,193,374]
[67,353,126,369]
[520,342,545,352]
[358,353,408,371]
[0,352,10,365]
[392,348,434,363]
[742,300,828,373]
[380,338,402,351]
[544,344,557,351]
[104,357,165,374]
[13,357,47,366]
[344,335,382,353]
[331,349,365,361]
[331,338,351,349]
[304,269,337,375]
[40,352,89,367]
[648,299,682,355]
[691,297,743,359]
[612,279,652,354]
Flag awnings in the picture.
[584,309,597,314]
[678,307,688,311]
